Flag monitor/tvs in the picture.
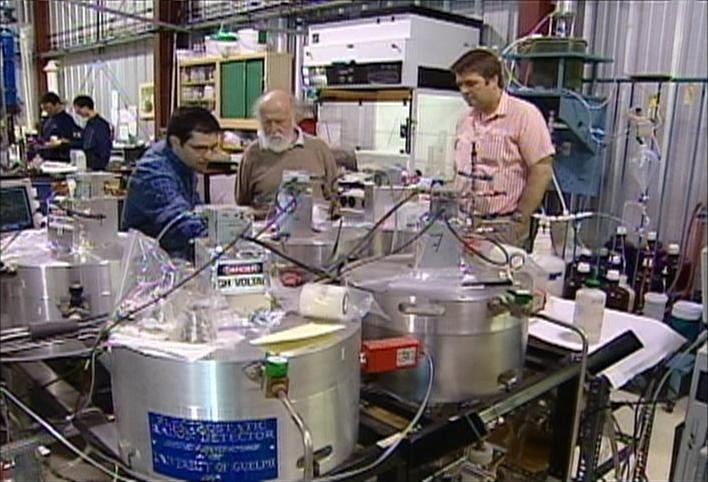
[0,177,37,238]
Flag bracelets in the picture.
[512,210,526,223]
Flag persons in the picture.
[450,49,557,252]
[120,108,221,262]
[234,89,339,219]
[29,93,75,162]
[62,95,113,170]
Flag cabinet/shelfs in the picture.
[175,48,295,135]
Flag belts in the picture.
[481,212,513,220]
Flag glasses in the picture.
[188,142,219,155]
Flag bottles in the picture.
[564,226,681,345]
